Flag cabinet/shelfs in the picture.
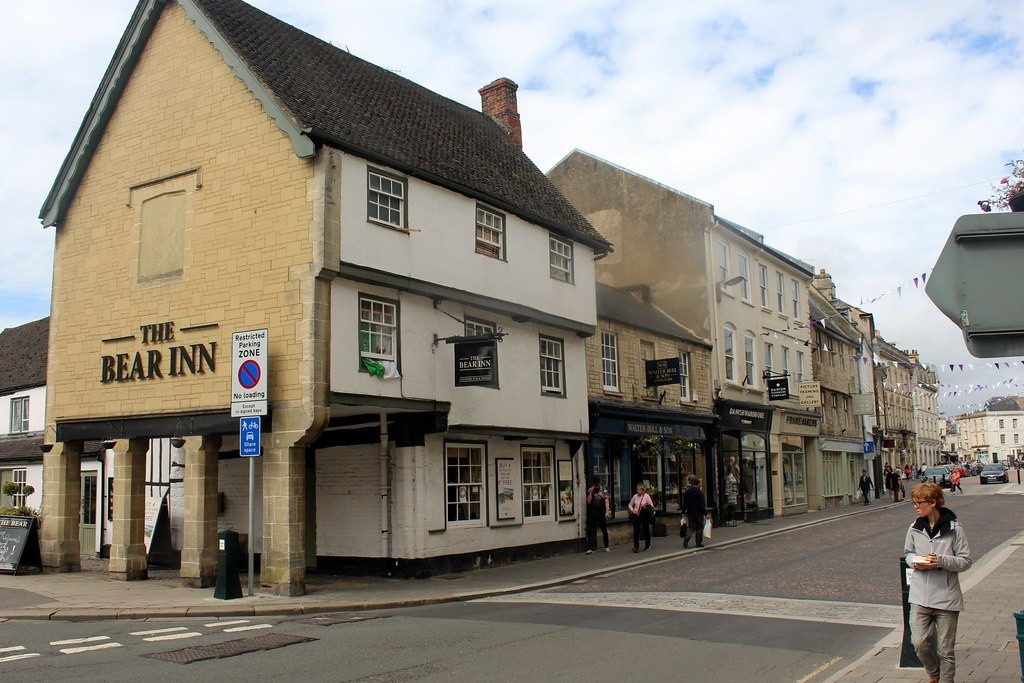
[640,456,689,510]
[594,455,621,510]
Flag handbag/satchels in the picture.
[703,517,712,538]
[855,489,863,500]
[679,516,687,538]
[628,512,638,523]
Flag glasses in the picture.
[911,501,929,507]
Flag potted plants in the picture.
[652,491,667,537]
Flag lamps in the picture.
[171,418,193,448]
[102,421,124,448]
[39,425,61,452]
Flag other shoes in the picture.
[696,542,704,547]
[633,547,639,553]
[585,549,594,554]
[929,674,939,683]
[605,547,610,552]
[644,545,651,551]
[683,540,688,547]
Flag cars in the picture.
[923,461,1009,488]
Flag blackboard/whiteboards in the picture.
[0,516,42,571]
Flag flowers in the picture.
[636,431,664,456]
[669,433,700,457]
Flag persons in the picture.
[680,478,710,547]
[627,483,655,553]
[723,456,755,507]
[884,458,1020,502]
[586,478,611,554]
[904,483,973,683]
[857,469,874,506]
